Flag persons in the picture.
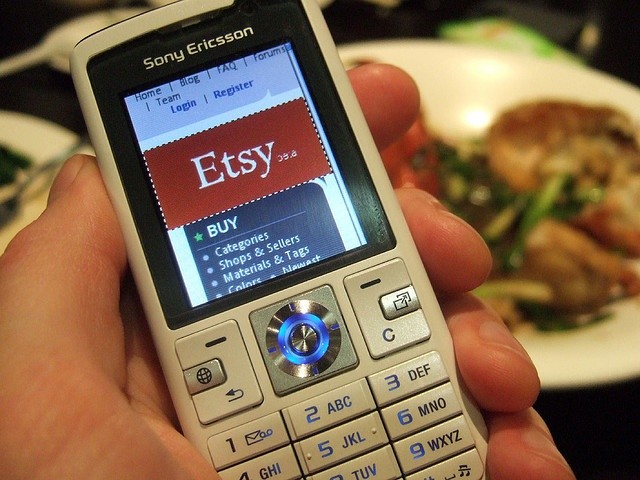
[1,63,580,479]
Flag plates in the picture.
[48,8,163,74]
[0,107,96,254]
[338,40,639,389]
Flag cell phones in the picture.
[68,0,502,479]
